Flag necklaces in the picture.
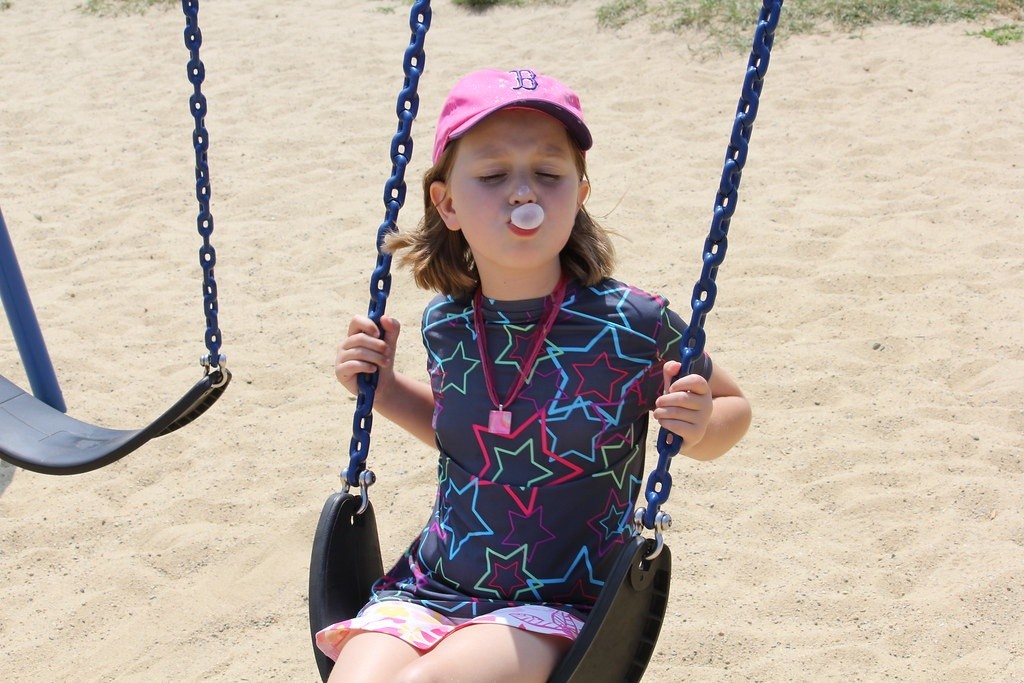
[473,263,572,434]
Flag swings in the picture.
[310,0,784,683]
[0,0,233,477]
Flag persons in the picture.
[315,68,751,683]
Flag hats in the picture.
[432,66,593,167]
